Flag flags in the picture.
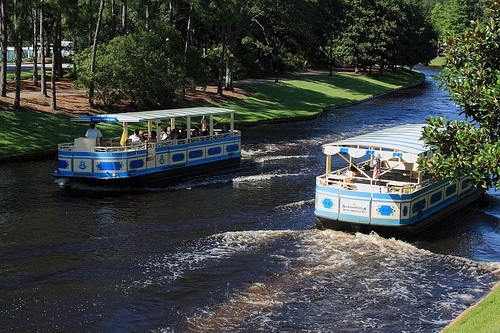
[372,157,380,180]
[120,131,128,147]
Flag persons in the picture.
[86,122,103,147]
[128,120,230,147]
[346,163,362,179]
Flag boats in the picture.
[52,107,241,179]
[315,125,486,228]
